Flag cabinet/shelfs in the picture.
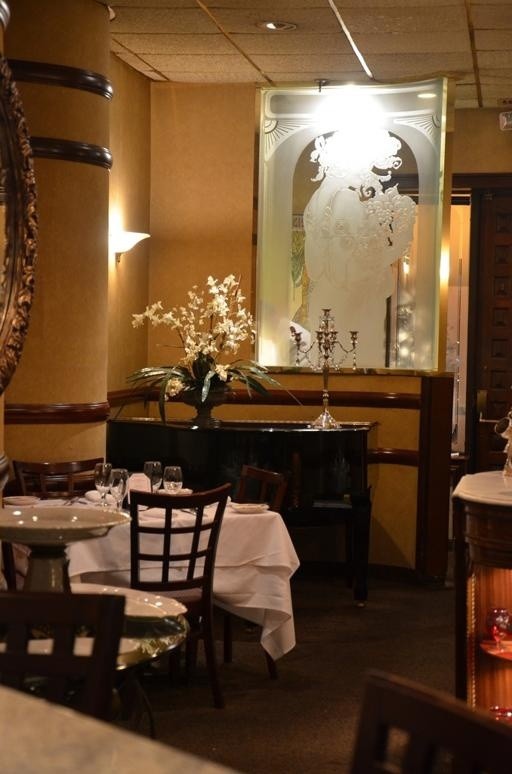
[104,417,379,614]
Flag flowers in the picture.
[125,272,284,429]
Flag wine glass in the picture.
[109,469,129,510]
[163,466,183,495]
[94,463,113,508]
[487,607,511,655]
[144,462,163,493]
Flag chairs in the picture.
[1,590,127,723]
[125,484,234,710]
[11,457,105,500]
[223,465,287,678]
[349,667,512,774]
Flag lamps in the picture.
[109,230,151,262]
[289,308,360,429]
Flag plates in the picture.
[3,496,40,506]
[143,507,179,519]
[85,490,118,504]
[231,503,270,514]
[70,580,188,624]
[0,508,132,544]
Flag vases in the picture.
[182,390,227,428]
